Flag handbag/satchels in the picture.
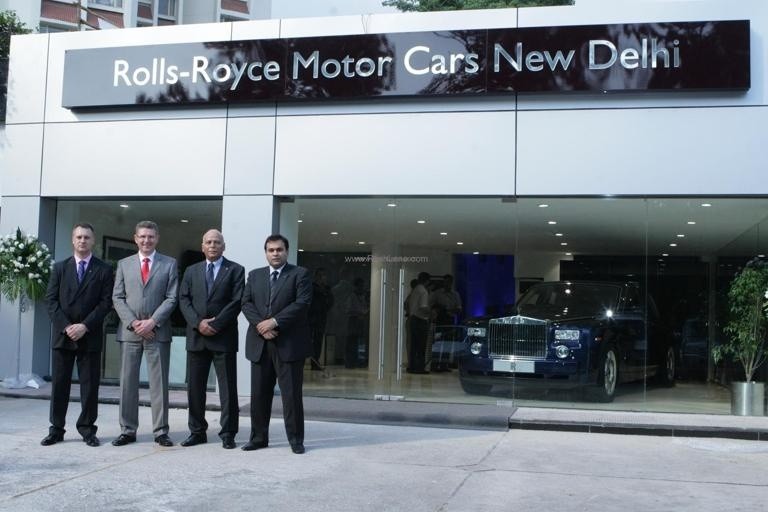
[303,338,315,356]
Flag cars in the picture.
[458,279,689,402]
[431,324,470,367]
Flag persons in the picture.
[402,270,464,375]
[111,220,179,447]
[40,220,114,446]
[179,227,246,449]
[307,264,369,373]
[239,230,314,455]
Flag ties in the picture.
[206,263,215,293]
[141,258,151,283]
[76,260,86,282]
[270,271,278,303]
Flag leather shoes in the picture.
[312,359,324,370]
[82,435,98,447]
[222,437,236,450]
[42,435,64,446]
[112,434,135,446]
[182,433,207,446]
[291,443,305,453]
[242,441,269,451]
[155,434,173,447]
[406,365,452,374]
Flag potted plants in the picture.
[707,256,768,417]
